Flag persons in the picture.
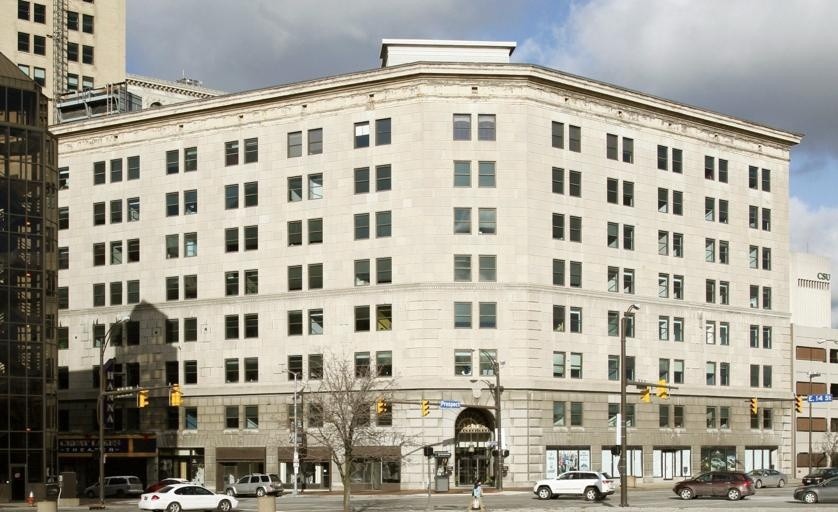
[476,480,481,508]
[471,483,482,511]
[227,471,235,485]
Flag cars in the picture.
[83,472,284,511]
[743,469,789,489]
[795,476,838,503]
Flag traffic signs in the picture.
[439,399,460,409]
[808,394,832,402]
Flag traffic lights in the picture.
[610,444,621,455]
[420,400,431,417]
[656,378,668,399]
[423,446,434,456]
[794,396,803,415]
[750,398,758,415]
[504,449,510,457]
[640,384,652,402]
[376,398,388,416]
[492,450,498,457]
[136,387,151,409]
[168,383,185,408]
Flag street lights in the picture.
[470,346,504,490]
[619,302,641,507]
[816,338,837,345]
[93,313,131,506]
[274,370,299,495]
[808,372,822,474]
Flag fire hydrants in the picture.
[28,489,36,506]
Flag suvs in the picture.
[534,470,616,504]
[672,472,756,502]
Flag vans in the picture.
[802,466,838,486]
